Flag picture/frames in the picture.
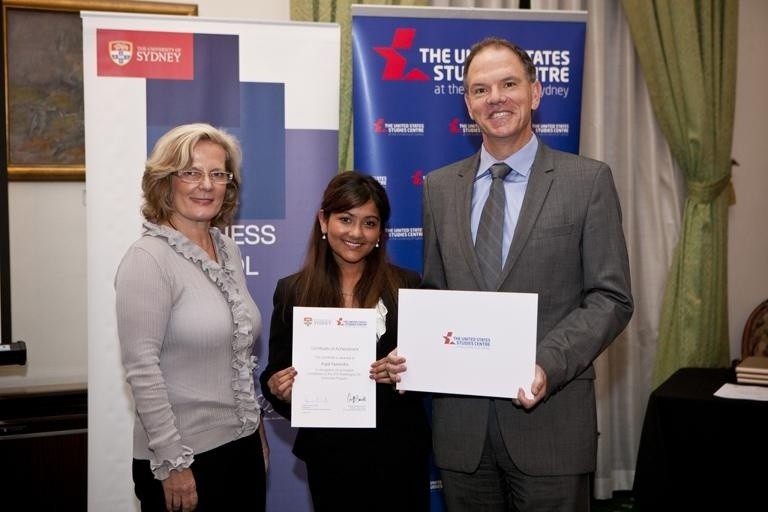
[1,0,198,181]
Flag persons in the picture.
[386,37,634,509]
[114,122,269,512]
[260,171,432,512]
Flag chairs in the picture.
[732,299,768,367]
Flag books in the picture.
[736,357,768,384]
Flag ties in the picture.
[474,163,513,293]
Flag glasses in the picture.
[171,171,233,185]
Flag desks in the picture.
[630,367,768,512]
